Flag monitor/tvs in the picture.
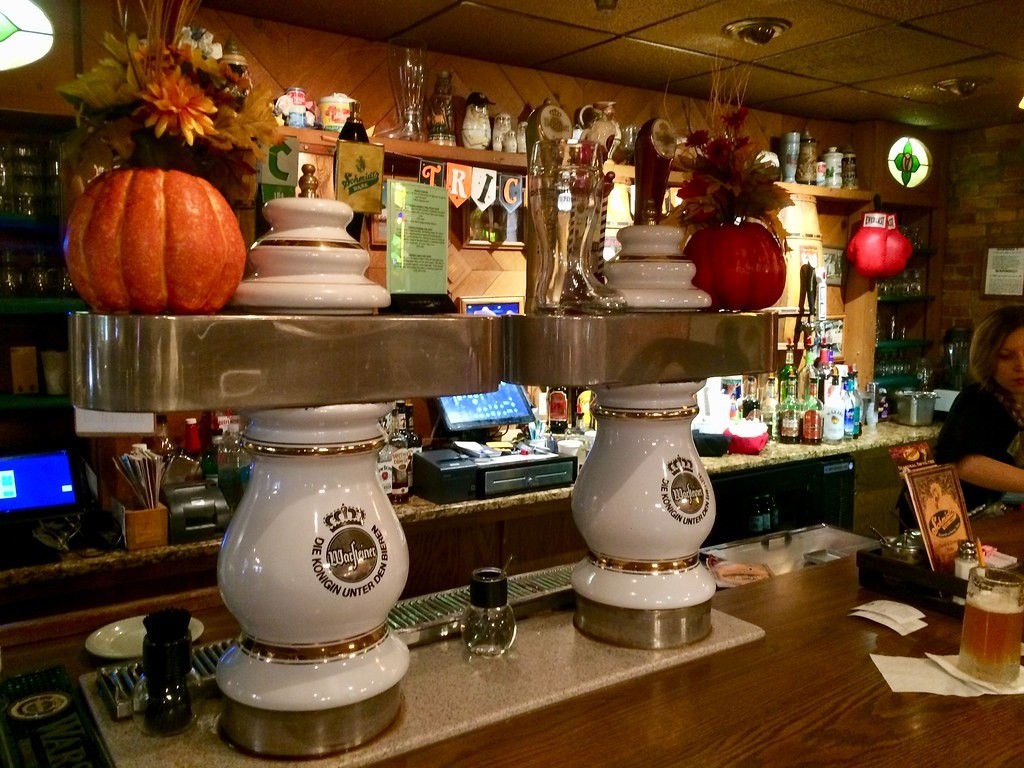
[436,381,536,431]
[0,449,82,519]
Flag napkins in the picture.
[834,647,1022,700]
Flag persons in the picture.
[933,307,1024,501]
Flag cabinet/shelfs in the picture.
[0,97,876,409]
[879,207,937,417]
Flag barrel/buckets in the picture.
[773,195,826,343]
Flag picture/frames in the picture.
[901,464,982,575]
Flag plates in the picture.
[85,615,205,658]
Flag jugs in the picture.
[578,101,622,154]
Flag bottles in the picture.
[428,67,456,145]
[550,386,569,433]
[460,569,520,661]
[956,566,1024,685]
[743,337,864,444]
[575,386,593,433]
[378,402,422,504]
[955,541,979,581]
[141,606,194,731]
[155,414,254,513]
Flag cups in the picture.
[373,46,422,142]
[530,141,626,313]
[42,352,68,394]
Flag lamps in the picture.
[867,132,947,203]
[931,78,981,97]
[723,17,793,47]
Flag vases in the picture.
[682,222,788,313]
[61,127,248,315]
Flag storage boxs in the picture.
[121,498,169,550]
[894,390,940,426]
[857,544,967,620]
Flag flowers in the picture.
[55,0,258,164]
[660,40,795,255]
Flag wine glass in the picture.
[872,227,927,376]
